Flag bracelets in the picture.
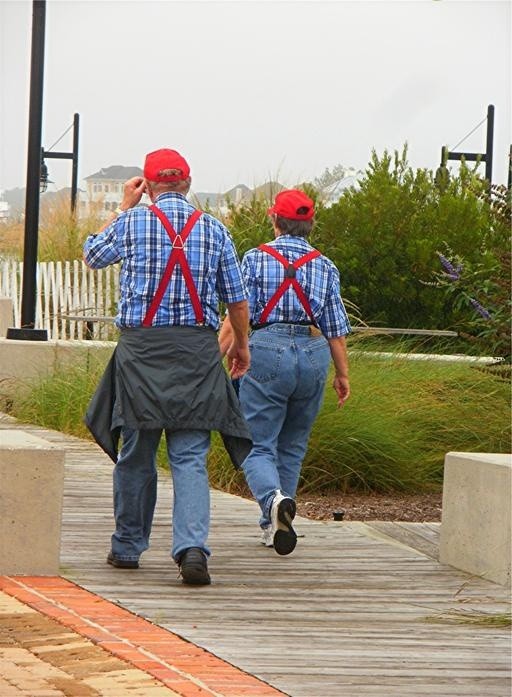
[112,205,127,217]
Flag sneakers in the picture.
[261,524,273,547]
[270,489,297,556]
[107,549,139,568]
[179,548,210,585]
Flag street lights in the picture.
[432,142,492,253]
[5,143,52,341]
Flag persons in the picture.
[80,147,254,586]
[215,187,354,557]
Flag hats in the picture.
[145,149,189,182]
[267,191,315,221]
